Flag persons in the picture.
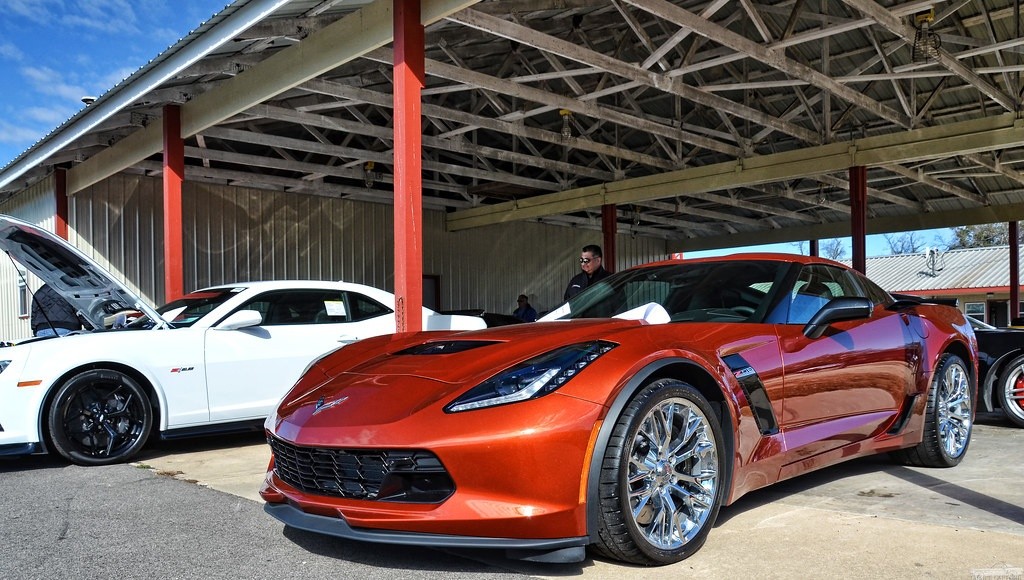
[512,294,537,325]
[564,245,628,319]
[30,284,94,337]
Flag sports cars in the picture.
[262,249,982,567]
[0,213,488,467]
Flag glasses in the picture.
[579,257,598,263]
[518,300,523,302]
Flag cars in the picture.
[963,315,1024,427]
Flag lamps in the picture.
[911,8,942,64]
[560,108,573,141]
[363,162,375,192]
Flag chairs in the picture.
[801,283,833,300]
[688,287,719,310]
[272,303,292,323]
